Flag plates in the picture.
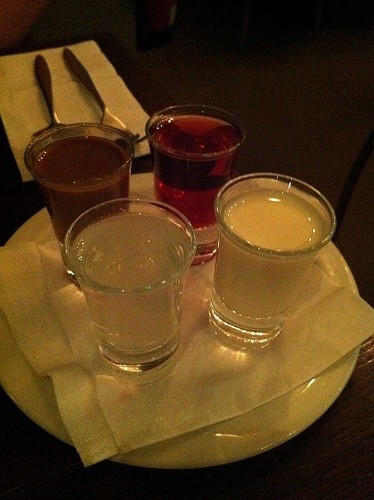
[0,173,361,467]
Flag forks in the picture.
[62,47,146,143]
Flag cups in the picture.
[146,104,246,265]
[25,121,135,284]
[63,197,196,372]
[207,173,336,352]
